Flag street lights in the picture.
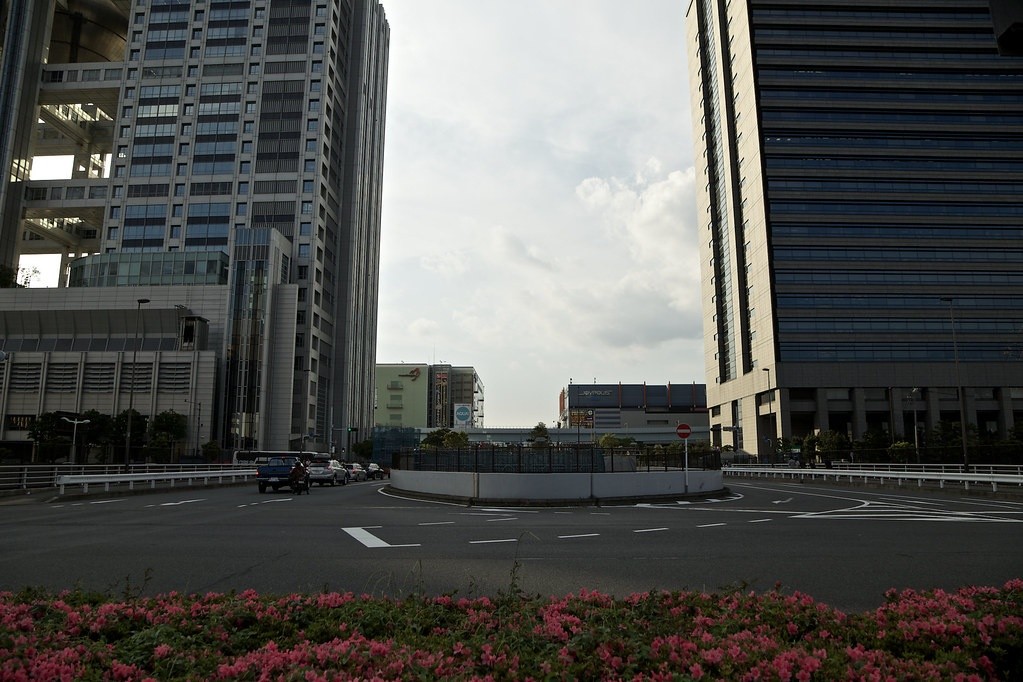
[762,367,774,468]
[61,417,90,475]
[939,295,971,474]
[126,298,151,474]
[588,409,593,498]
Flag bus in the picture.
[231,450,319,480]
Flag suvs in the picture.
[361,462,384,480]
[305,459,349,486]
[342,462,367,482]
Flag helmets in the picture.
[295,461,301,466]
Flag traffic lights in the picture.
[347,428,358,432]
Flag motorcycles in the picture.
[289,467,309,495]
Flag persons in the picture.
[288,460,311,494]
[16,452,33,488]
[788,457,796,479]
[48,459,60,487]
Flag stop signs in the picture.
[676,423,691,439]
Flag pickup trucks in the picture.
[255,456,311,493]
